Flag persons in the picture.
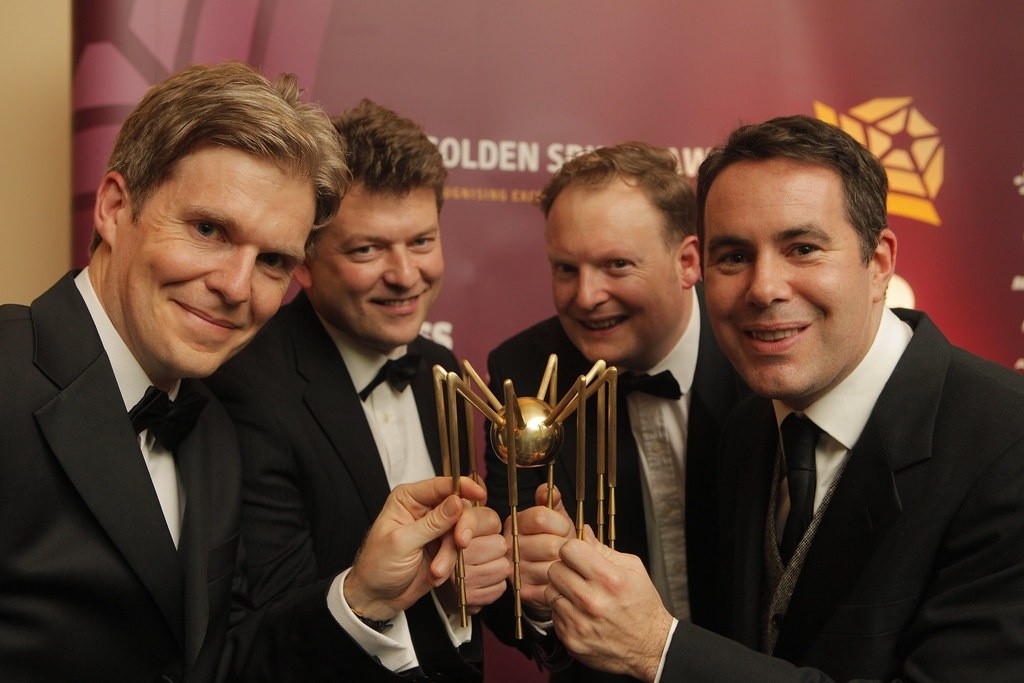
[0,63,487,683]
[206,111,510,683]
[545,114,1024,682]
[472,142,756,683]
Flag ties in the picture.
[779,412,823,568]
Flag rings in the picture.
[550,593,565,608]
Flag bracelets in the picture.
[346,603,399,635]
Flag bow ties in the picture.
[359,354,422,402]
[127,385,210,453]
[615,369,682,401]
